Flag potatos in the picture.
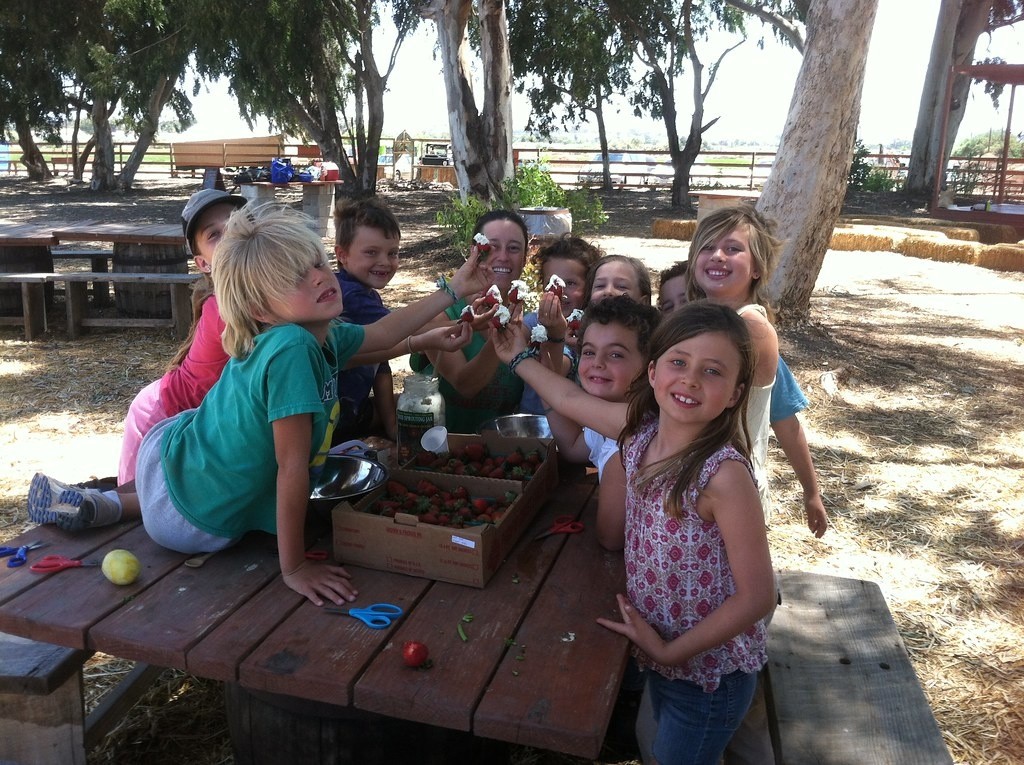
[101,550,141,584]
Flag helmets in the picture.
[181,188,248,239]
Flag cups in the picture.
[420,426,450,455]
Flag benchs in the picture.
[761,571,954,764]
[45,269,203,341]
[51,249,114,306]
[1,631,165,765]
[0,273,53,340]
[52,158,77,176]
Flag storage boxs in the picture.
[329,431,560,591]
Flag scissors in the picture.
[324,603,403,629]
[0,539,50,568]
[534,514,584,540]
[304,550,328,560]
[30,555,101,572]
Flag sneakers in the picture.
[25,471,119,533]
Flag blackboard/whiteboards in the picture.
[202,167,225,191]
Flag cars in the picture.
[648,158,714,191]
[577,153,655,187]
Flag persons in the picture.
[116,189,252,486]
[492,200,830,764]
[420,209,525,430]
[330,200,500,445]
[25,197,495,606]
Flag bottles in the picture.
[395,374,445,465]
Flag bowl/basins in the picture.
[476,413,586,475]
[307,453,390,518]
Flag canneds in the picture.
[395,374,445,467]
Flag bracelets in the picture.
[435,276,460,304]
[406,335,416,355]
[544,406,553,415]
[547,336,566,343]
[506,347,543,376]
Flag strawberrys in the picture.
[458,232,585,344]
[401,640,434,670]
[414,441,543,481]
[370,479,516,528]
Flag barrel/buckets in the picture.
[0,244,54,318]
[225,680,450,765]
[113,241,189,319]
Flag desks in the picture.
[0,455,631,765]
[0,223,58,318]
[687,187,762,230]
[52,221,190,318]
[233,178,345,219]
